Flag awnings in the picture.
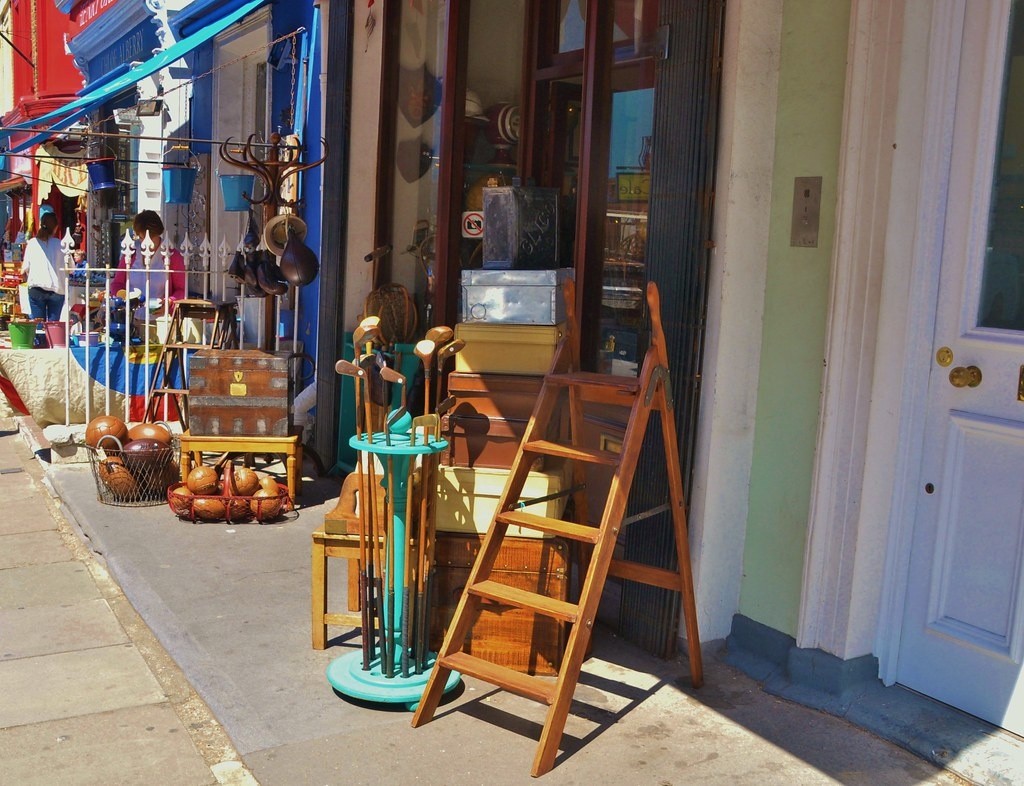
[1,0,271,162]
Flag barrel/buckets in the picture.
[6,320,37,349]
[83,142,117,190]
[43,321,72,348]
[159,145,201,204]
[215,149,255,211]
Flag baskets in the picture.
[84,420,182,506]
[168,480,290,523]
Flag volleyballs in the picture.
[85,416,129,456]
[168,465,291,522]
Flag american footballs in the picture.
[127,423,173,442]
[99,456,138,500]
[123,441,173,467]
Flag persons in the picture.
[72,249,90,283]
[21,211,76,324]
[98,208,186,320]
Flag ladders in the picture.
[410,278,704,778]
[141,300,239,434]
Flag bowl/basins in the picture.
[75,332,99,347]
[144,298,162,316]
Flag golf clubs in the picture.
[333,307,467,678]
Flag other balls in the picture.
[147,469,168,495]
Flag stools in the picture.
[311,517,425,651]
[179,422,305,508]
